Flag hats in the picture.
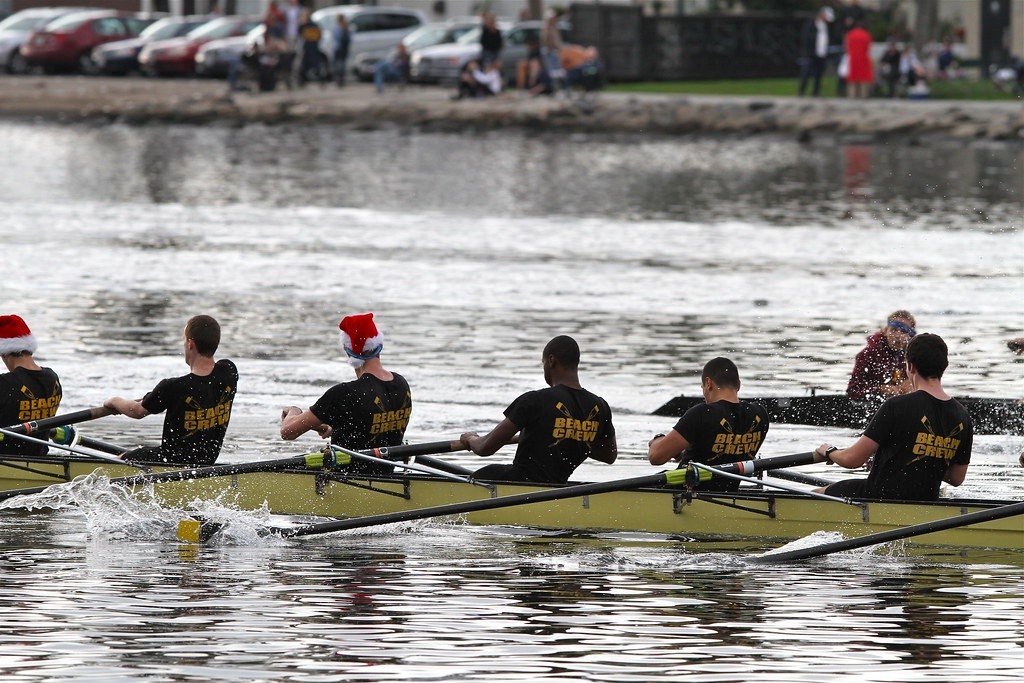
[340,313,383,367]
[0,314,37,355]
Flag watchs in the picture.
[826,446,837,465]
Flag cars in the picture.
[196,7,429,78]
[350,20,508,80]
[828,43,981,68]
[0,6,155,75]
[409,17,571,85]
[91,16,213,78]
[138,15,268,77]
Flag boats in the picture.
[0,453,1024,547]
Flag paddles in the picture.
[676,451,836,486]
[312,425,474,479]
[1,398,145,443]
[1,434,520,504]
[49,427,134,458]
[763,501,1023,564]
[176,447,846,545]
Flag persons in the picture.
[798,6,956,98]
[810,332,973,500]
[848,310,915,398]
[104,315,239,466]
[451,7,600,101]
[648,358,770,491]
[242,7,351,91]
[369,43,409,92]
[0,315,62,455]
[460,335,617,484]
[280,313,412,473]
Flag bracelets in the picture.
[654,433,665,439]
